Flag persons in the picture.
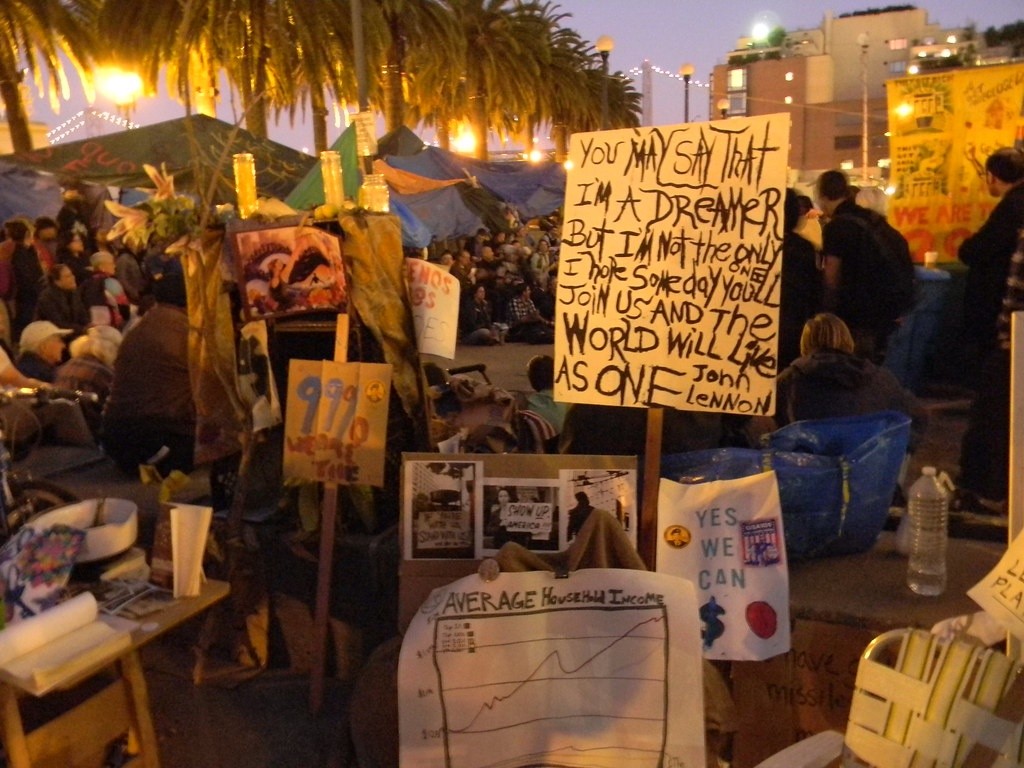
[434,193,576,352]
[1,154,219,447]
[999,210,1023,407]
[565,404,709,452]
[812,170,916,368]
[523,356,574,453]
[208,445,252,561]
[765,313,930,432]
[956,146,1024,518]
[721,187,819,448]
[100,271,205,477]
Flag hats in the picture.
[19,319,72,355]
[56,208,79,226]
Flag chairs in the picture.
[837,627,1024,768]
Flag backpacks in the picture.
[834,211,915,316]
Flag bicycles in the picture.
[1,385,99,541]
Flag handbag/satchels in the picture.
[632,413,917,565]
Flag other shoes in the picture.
[949,482,1003,513]
[251,666,356,711]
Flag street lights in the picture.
[680,63,696,123]
[857,32,871,179]
[717,98,731,120]
[596,35,613,130]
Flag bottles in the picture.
[906,466,948,597]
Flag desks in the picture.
[1,541,233,767]
[731,528,1007,768]
